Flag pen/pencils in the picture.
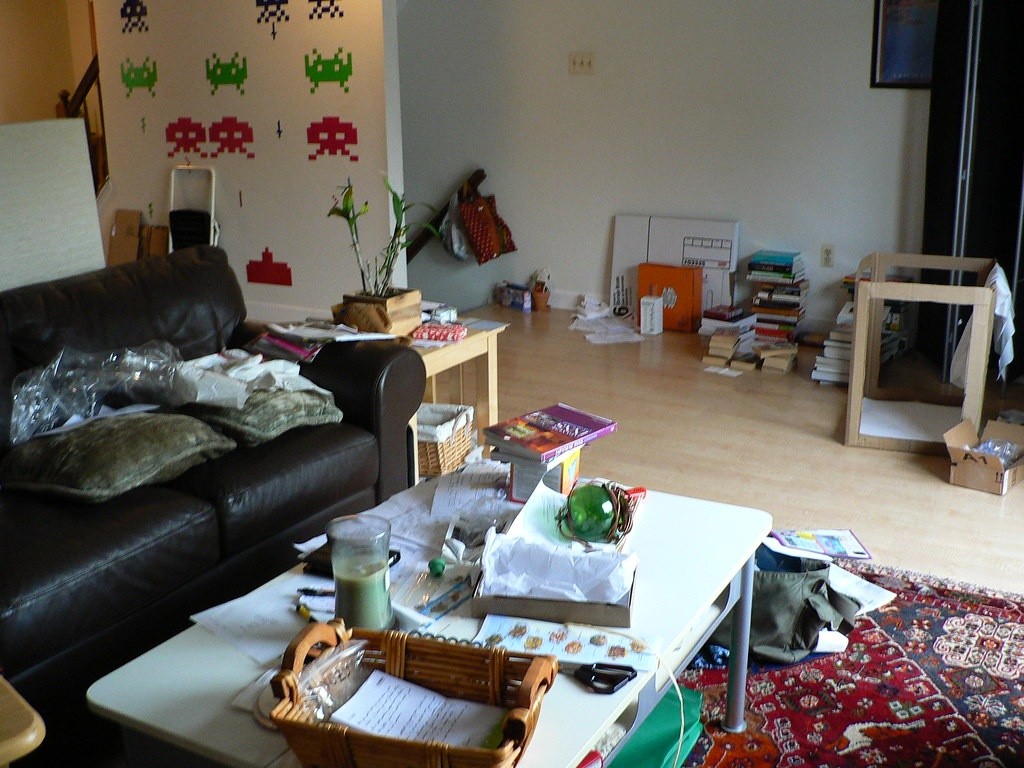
[296,604,317,623]
[297,587,335,598]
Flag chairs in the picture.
[168,165,221,254]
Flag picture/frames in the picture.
[870,0,942,89]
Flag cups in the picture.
[325,514,395,630]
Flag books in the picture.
[812,271,915,386]
[482,402,618,473]
[698,248,810,375]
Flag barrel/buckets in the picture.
[532,290,551,311]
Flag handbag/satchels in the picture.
[459,180,518,267]
[708,542,863,665]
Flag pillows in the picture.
[186,386,344,447]
[0,412,236,501]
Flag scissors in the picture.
[556,658,636,694]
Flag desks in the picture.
[85,479,773,767]
[389,317,511,487]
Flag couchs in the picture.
[0,246,426,709]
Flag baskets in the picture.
[417,403,473,478]
[267,618,558,768]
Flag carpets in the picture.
[676,557,1024,768]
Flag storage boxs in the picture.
[108,208,168,266]
[942,416,1024,496]
[637,263,703,333]
[471,571,638,628]
[508,447,581,504]
[502,280,531,313]
[609,214,739,326]
[640,296,663,335]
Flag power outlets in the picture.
[820,244,834,268]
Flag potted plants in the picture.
[327,176,441,337]
